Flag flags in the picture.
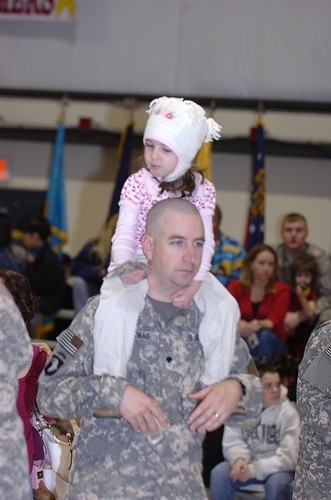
[197,102,215,181]
[56,328,84,356]
[242,103,267,252]
[44,97,68,256]
[97,105,135,225]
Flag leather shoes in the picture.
[92,404,120,420]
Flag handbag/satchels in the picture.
[31,397,73,500]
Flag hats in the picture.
[142,95,222,183]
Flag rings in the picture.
[214,412,220,419]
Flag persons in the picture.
[279,254,320,365]
[209,363,302,500]
[210,203,248,287]
[293,320,331,500]
[268,212,331,358]
[65,213,119,316]
[37,197,261,500]
[0,207,82,500]
[92,97,248,419]
[227,243,291,369]
